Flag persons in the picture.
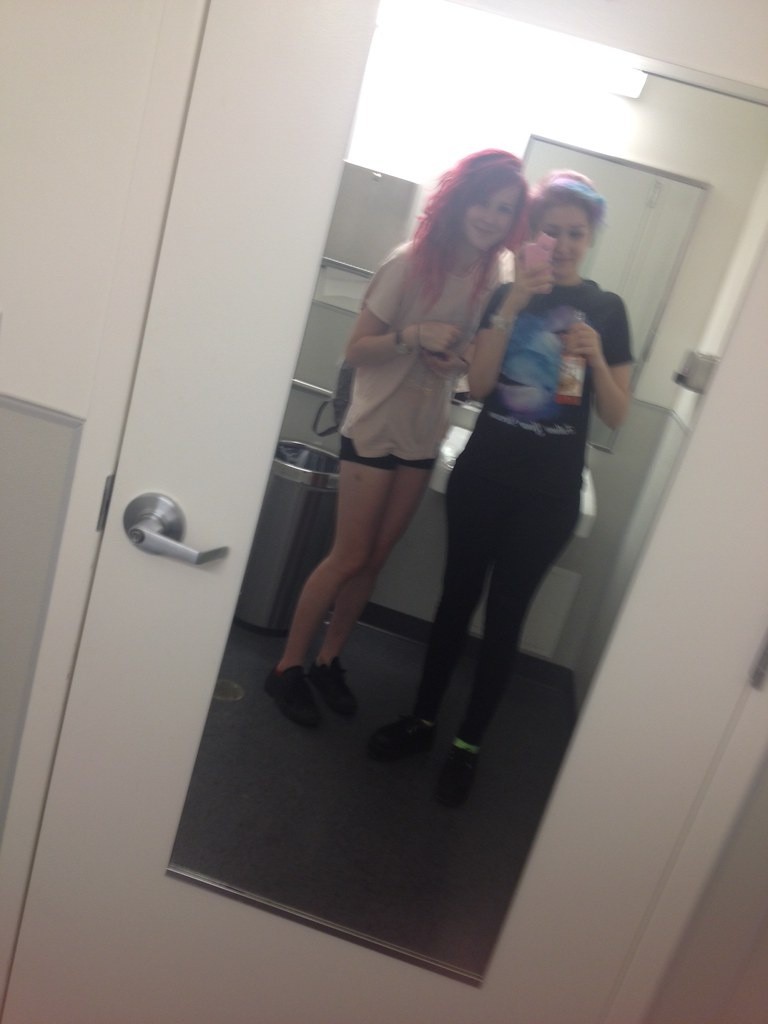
[354,164,635,809]
[257,150,531,726]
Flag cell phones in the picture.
[524,243,551,294]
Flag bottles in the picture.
[554,311,587,407]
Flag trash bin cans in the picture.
[235,440,339,628]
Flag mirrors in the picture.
[166,0,767,997]
[446,133,717,453]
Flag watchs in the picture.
[394,330,409,355]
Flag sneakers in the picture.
[368,711,435,760]
[308,654,360,718]
[261,662,323,731]
[433,741,480,809]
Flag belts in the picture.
[484,410,581,436]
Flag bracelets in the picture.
[488,313,517,330]
